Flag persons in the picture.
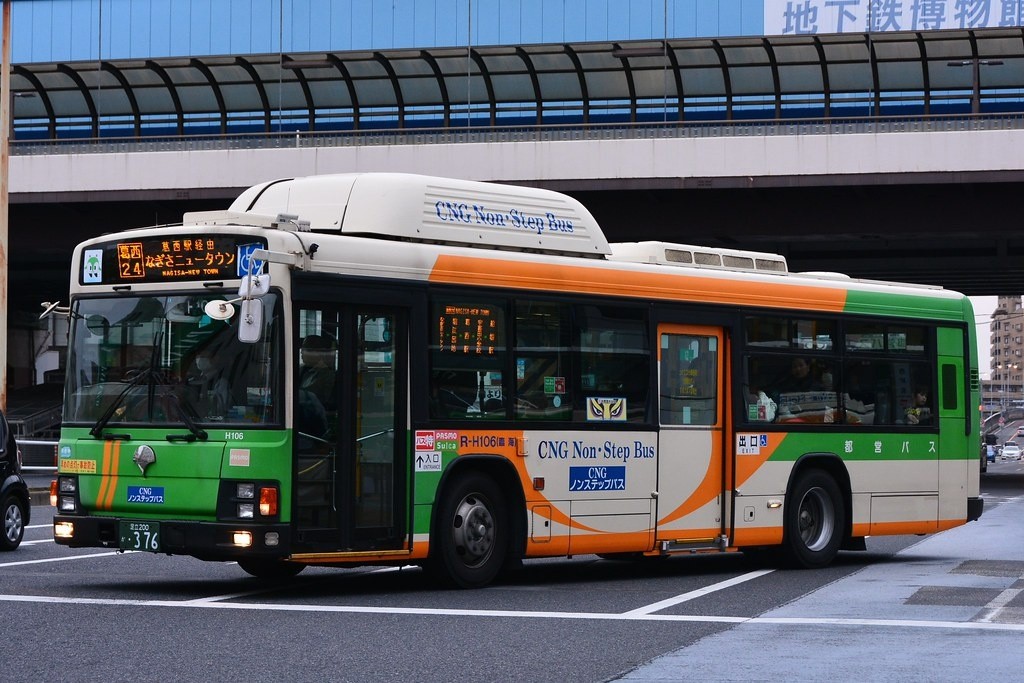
[777,357,822,394]
[895,388,930,425]
[293,335,337,407]
[187,346,226,390]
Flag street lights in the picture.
[994,362,1018,407]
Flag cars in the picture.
[0,411,31,553]
[980,432,1024,472]
[1017,426,1024,436]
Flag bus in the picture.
[39,172,984,591]
